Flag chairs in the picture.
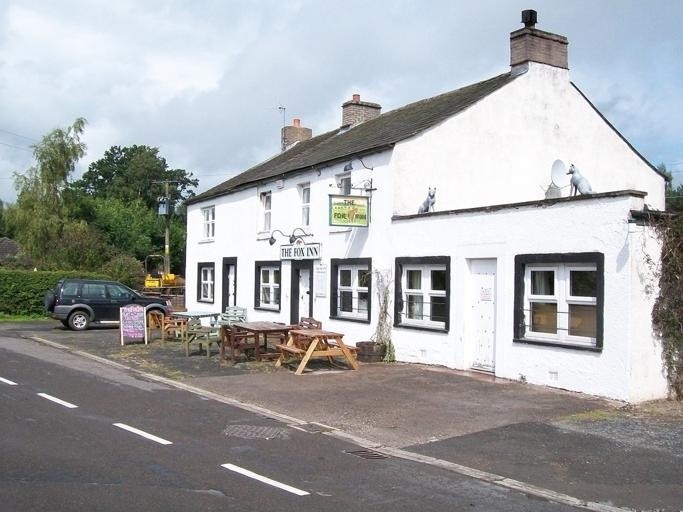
[148,305,322,373]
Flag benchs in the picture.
[274,342,360,354]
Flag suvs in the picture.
[44,278,169,331]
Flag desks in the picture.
[288,329,359,375]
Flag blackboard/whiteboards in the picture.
[120,304,147,338]
